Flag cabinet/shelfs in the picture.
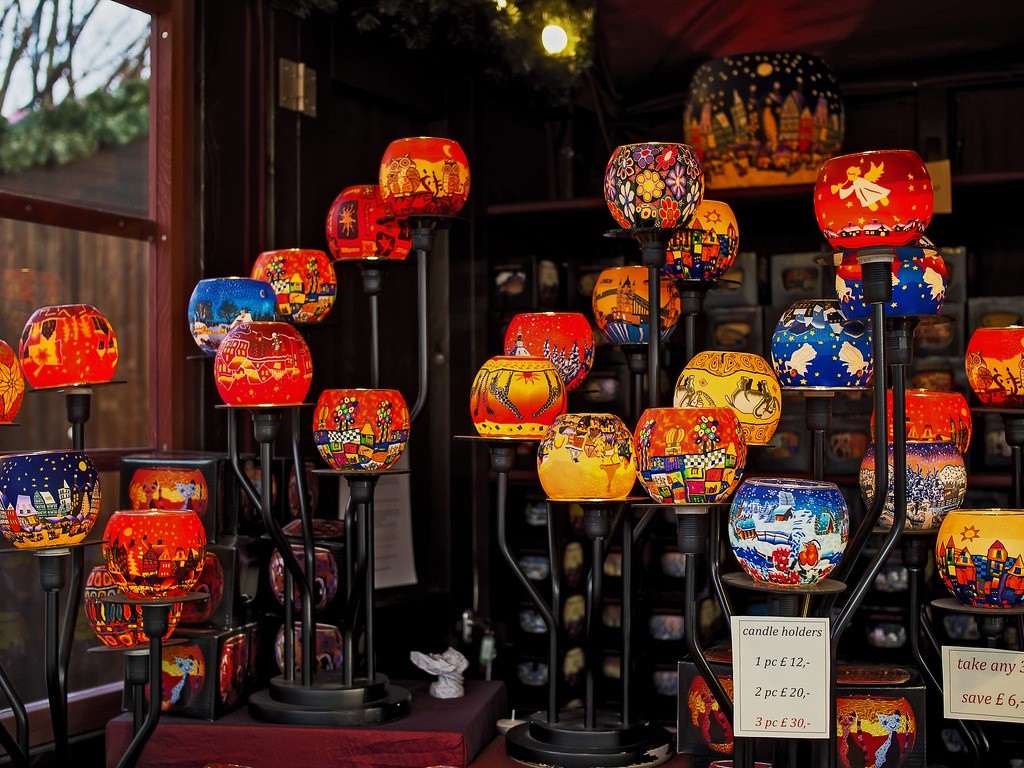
[472,169,1024,723]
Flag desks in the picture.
[105,681,504,768]
